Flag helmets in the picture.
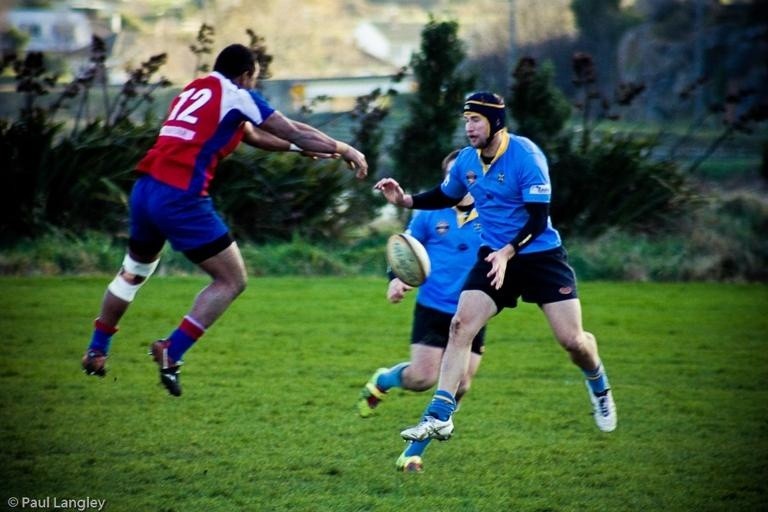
[461,91,507,149]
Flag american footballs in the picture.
[386,234,433,287]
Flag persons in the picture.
[374,93,618,443]
[359,149,485,474]
[82,43,369,396]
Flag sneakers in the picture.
[394,453,425,474]
[81,347,107,377]
[149,338,182,397]
[584,376,618,432]
[357,367,389,418]
[400,415,456,442]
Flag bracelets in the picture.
[288,143,303,153]
